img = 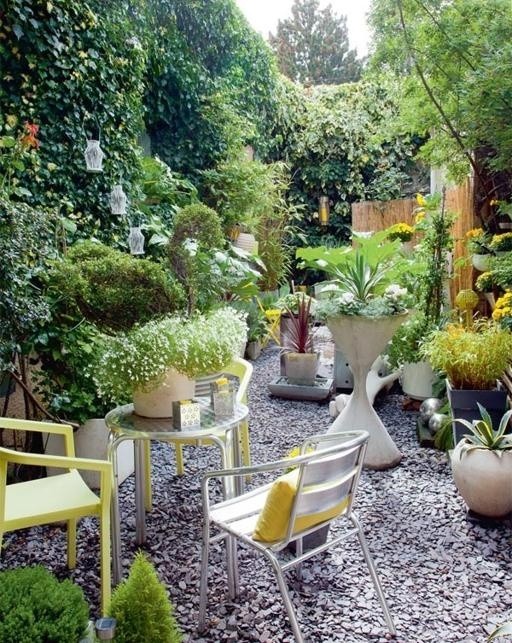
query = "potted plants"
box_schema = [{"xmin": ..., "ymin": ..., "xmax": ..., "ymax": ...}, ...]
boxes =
[
  {"xmin": 418, "ymin": 313, "xmax": 512, "ymax": 446},
  {"xmin": 381, "ymin": 319, "xmax": 440, "ymax": 401},
  {"xmin": 163, "ymin": 203, "xmax": 225, "ymax": 396},
  {"xmin": 93, "ymin": 307, "xmax": 231, "ymax": 418},
  {"xmin": 246, "ymin": 311, "xmax": 271, "ymax": 359},
  {"xmin": 467, "ymin": 234, "xmax": 492, "ymax": 271},
  {"xmin": 277, "ymin": 291, "xmax": 317, "ymax": 376},
  {"xmin": 41, "ymin": 325, "xmax": 134, "ymax": 489},
  {"xmin": 283, "ymin": 296, "xmax": 317, "ymax": 385},
  {"xmin": 452, "ymin": 401, "xmax": 512, "ymax": 518},
  {"xmin": 295, "ymin": 230, "xmax": 409, "ymax": 470},
  {"xmin": 232, "ymin": 209, "xmax": 262, "ymax": 251}
]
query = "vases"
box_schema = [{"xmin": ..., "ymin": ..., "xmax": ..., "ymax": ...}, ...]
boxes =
[{"xmin": 268, "ymin": 375, "xmax": 334, "ymax": 400}]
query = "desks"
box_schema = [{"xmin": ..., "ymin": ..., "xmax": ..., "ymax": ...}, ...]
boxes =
[{"xmin": 105, "ymin": 398, "xmax": 249, "ymax": 601}]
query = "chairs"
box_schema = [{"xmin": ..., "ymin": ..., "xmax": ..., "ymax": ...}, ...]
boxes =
[
  {"xmin": 198, "ymin": 431, "xmax": 396, "ymax": 643},
  {"xmin": 0, "ymin": 417, "xmax": 113, "ymax": 618},
  {"xmin": 144, "ymin": 357, "xmax": 254, "ymax": 511}
]
